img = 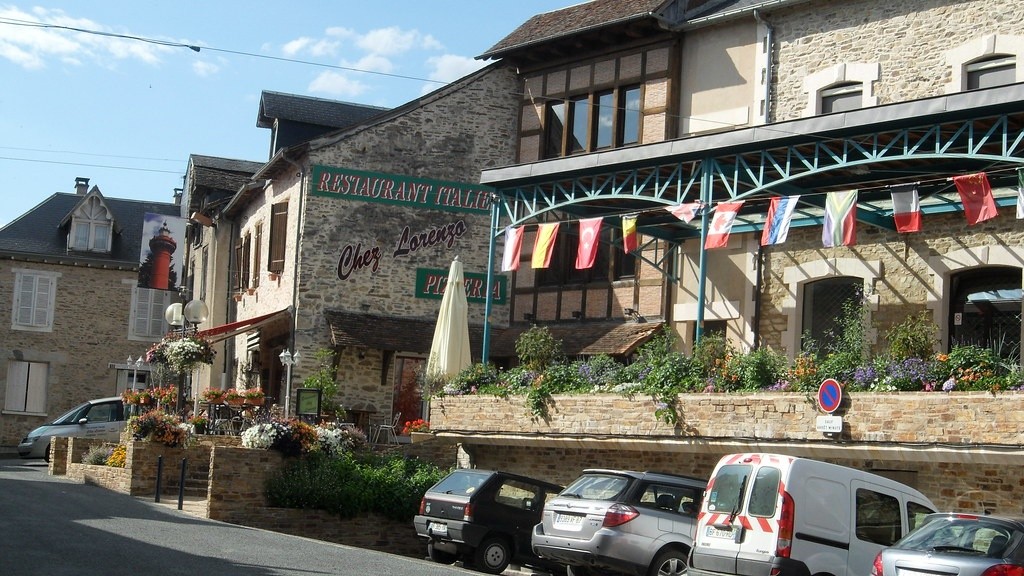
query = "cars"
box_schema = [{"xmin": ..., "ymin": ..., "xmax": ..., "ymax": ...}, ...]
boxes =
[
  {"xmin": 869, "ymin": 512, "xmax": 1024, "ymax": 576},
  {"xmin": 17, "ymin": 397, "xmax": 131, "ymax": 463},
  {"xmin": 414, "ymin": 469, "xmax": 566, "ymax": 575},
  {"xmin": 532, "ymin": 469, "xmax": 709, "ymax": 576}
]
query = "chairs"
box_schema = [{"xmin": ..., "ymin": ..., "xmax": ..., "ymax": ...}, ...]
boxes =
[
  {"xmin": 988, "ymin": 536, "xmax": 1008, "ymax": 554},
  {"xmin": 658, "ymin": 494, "xmax": 674, "ymax": 510},
  {"xmin": 372, "ymin": 412, "xmax": 402, "ymax": 445}
]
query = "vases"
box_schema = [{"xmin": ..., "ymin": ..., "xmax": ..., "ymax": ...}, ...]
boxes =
[
  {"xmin": 247, "ymin": 289, "xmax": 255, "ymax": 296},
  {"xmin": 228, "ymin": 399, "xmax": 244, "ymax": 405},
  {"xmin": 234, "ymin": 295, "xmax": 241, "ymax": 302},
  {"xmin": 195, "ymin": 424, "xmax": 204, "ymax": 434},
  {"xmin": 248, "ymin": 398, "xmax": 265, "ymax": 405},
  {"xmin": 268, "ymin": 274, "xmax": 277, "ymax": 280},
  {"xmin": 206, "ymin": 398, "xmax": 223, "ymax": 404},
  {"xmin": 140, "ymin": 397, "xmax": 149, "ymax": 404}
]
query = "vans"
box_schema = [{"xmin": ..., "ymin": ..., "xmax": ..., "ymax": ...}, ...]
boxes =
[{"xmin": 687, "ymin": 453, "xmax": 958, "ymax": 576}]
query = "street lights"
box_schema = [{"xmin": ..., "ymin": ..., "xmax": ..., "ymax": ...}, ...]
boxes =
[
  {"xmin": 127, "ymin": 355, "xmax": 144, "ymax": 393},
  {"xmin": 165, "ymin": 299, "xmax": 208, "ymax": 412},
  {"xmin": 279, "ymin": 348, "xmax": 302, "ymax": 418}
]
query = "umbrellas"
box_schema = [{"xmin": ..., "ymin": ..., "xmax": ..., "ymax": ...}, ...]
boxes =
[{"xmin": 425, "ymin": 255, "xmax": 471, "ymax": 377}]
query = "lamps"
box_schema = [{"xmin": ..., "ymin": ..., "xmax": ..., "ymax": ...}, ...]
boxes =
[
  {"xmin": 624, "ymin": 307, "xmax": 646, "ymax": 324},
  {"xmin": 191, "ymin": 212, "xmax": 215, "ymax": 228},
  {"xmin": 572, "ymin": 311, "xmax": 587, "ymax": 322},
  {"xmin": 524, "ymin": 313, "xmax": 536, "ymax": 324}
]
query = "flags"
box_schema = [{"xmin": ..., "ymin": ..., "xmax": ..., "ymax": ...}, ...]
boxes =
[
  {"xmin": 704, "ymin": 202, "xmax": 743, "ymax": 250},
  {"xmin": 953, "ymin": 172, "xmax": 998, "ymax": 226},
  {"xmin": 622, "ymin": 215, "xmax": 637, "ymax": 254},
  {"xmin": 575, "ymin": 217, "xmax": 602, "ymax": 269},
  {"xmin": 501, "ymin": 224, "xmax": 524, "ymax": 272},
  {"xmin": 890, "ymin": 184, "xmax": 922, "ymax": 234},
  {"xmin": 530, "ymin": 222, "xmax": 559, "ymax": 268},
  {"xmin": 1016, "ymin": 168, "xmax": 1024, "ymax": 219},
  {"xmin": 761, "ymin": 197, "xmax": 799, "ymax": 246},
  {"xmin": 663, "ymin": 203, "xmax": 705, "ymax": 224},
  {"xmin": 821, "ymin": 189, "xmax": 858, "ymax": 248}
]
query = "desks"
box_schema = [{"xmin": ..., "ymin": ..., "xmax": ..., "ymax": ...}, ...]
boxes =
[{"xmin": 346, "ymin": 409, "xmax": 376, "ymax": 443}]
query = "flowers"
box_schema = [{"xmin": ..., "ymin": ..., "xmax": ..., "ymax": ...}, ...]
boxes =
[
  {"xmin": 239, "ymin": 421, "xmax": 345, "ymax": 456},
  {"xmin": 121, "ymin": 384, "xmax": 208, "ymax": 447},
  {"xmin": 146, "ymin": 330, "xmax": 217, "ymax": 377},
  {"xmin": 81, "ymin": 443, "xmax": 127, "ymax": 468},
  {"xmin": 403, "ymin": 277, "xmax": 1024, "ymax": 437},
  {"xmin": 202, "ymin": 387, "xmax": 265, "ymax": 401}
]
